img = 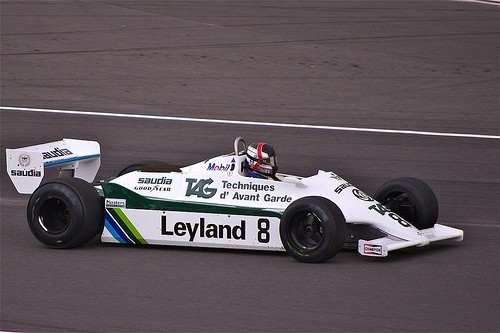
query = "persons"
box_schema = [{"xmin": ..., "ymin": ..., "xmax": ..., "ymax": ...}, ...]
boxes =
[{"xmin": 245, "ymin": 142, "xmax": 281, "ymax": 181}]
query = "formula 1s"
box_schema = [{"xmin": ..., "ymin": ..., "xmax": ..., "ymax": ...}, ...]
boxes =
[{"xmin": 5, "ymin": 136, "xmax": 465, "ymax": 265}]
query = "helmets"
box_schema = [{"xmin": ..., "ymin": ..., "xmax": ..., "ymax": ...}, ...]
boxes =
[{"xmin": 246, "ymin": 142, "xmax": 279, "ymax": 176}]
